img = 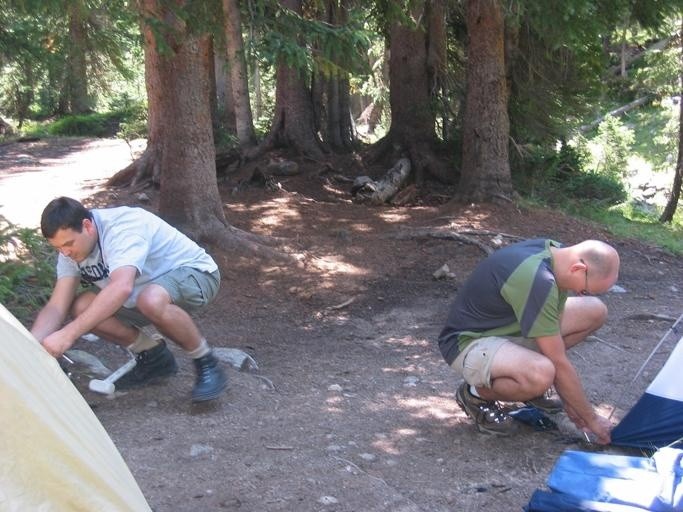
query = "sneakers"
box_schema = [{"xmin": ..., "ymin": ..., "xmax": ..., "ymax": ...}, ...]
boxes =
[
  {"xmin": 113, "ymin": 340, "xmax": 178, "ymax": 392},
  {"xmin": 455, "ymin": 381, "xmax": 520, "ymax": 436},
  {"xmin": 524, "ymin": 392, "xmax": 564, "ymax": 414},
  {"xmin": 190, "ymin": 350, "xmax": 228, "ymax": 401}
]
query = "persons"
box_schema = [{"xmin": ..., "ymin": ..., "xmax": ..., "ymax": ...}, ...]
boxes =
[
  {"xmin": 28, "ymin": 197, "xmax": 232, "ymax": 403},
  {"xmin": 438, "ymin": 239, "xmax": 620, "ymax": 446}
]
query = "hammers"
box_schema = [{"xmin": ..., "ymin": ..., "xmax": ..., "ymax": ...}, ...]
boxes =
[{"xmin": 80, "ymin": 359, "xmax": 138, "ymax": 395}]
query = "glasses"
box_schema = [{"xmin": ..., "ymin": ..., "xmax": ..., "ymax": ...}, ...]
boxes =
[{"xmin": 580, "ymin": 259, "xmax": 589, "ymax": 296}]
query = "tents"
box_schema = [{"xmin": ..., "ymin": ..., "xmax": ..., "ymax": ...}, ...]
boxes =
[
  {"xmin": 0, "ymin": 294, "xmax": 153, "ymax": 512},
  {"xmin": 597, "ymin": 336, "xmax": 683, "ymax": 449}
]
